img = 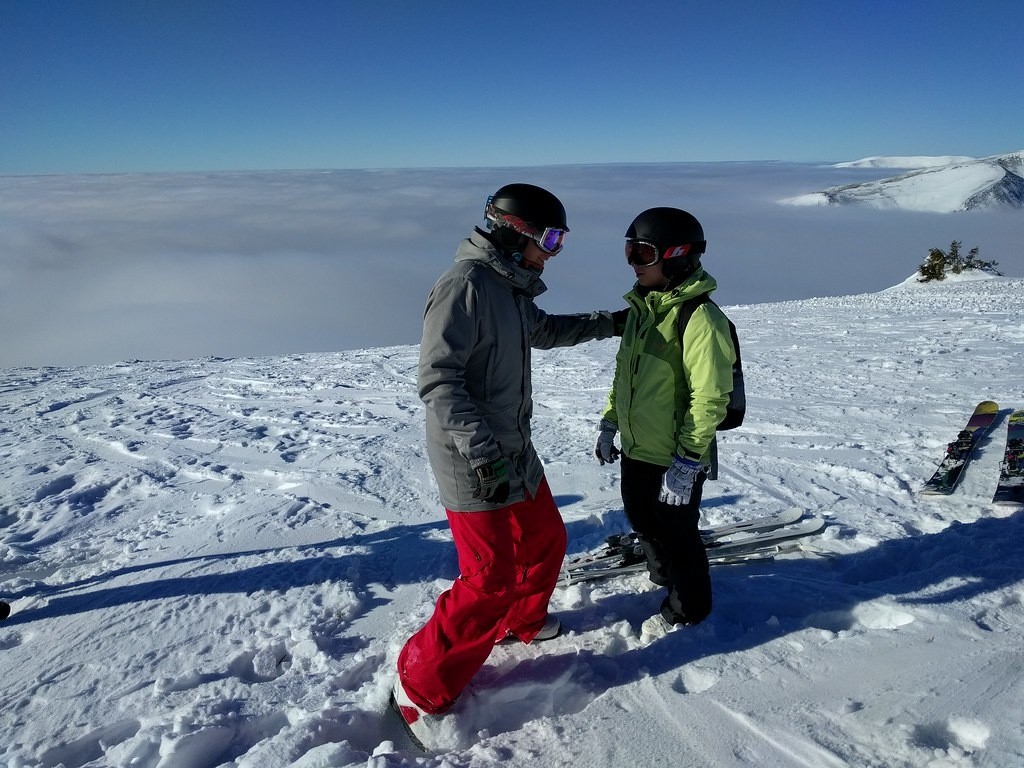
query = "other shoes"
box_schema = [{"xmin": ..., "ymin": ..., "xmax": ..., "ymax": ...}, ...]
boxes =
[
  {"xmin": 635, "ymin": 579, "xmax": 663, "ymax": 594},
  {"xmin": 390, "ymin": 673, "xmax": 444, "ymax": 752},
  {"xmin": 637, "ymin": 610, "xmax": 674, "ymax": 643},
  {"xmin": 495, "ymin": 614, "xmax": 561, "ymax": 645}
]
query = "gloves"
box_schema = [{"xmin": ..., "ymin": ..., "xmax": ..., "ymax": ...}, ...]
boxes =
[
  {"xmin": 473, "ymin": 457, "xmax": 511, "ymax": 505},
  {"xmin": 611, "ymin": 307, "xmax": 632, "ymax": 337},
  {"xmin": 594, "ymin": 419, "xmax": 620, "ymax": 466},
  {"xmin": 658, "ymin": 452, "xmax": 702, "ymax": 506}
]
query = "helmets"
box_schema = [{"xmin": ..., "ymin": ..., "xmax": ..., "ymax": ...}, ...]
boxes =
[
  {"xmin": 487, "ymin": 183, "xmax": 570, "ymax": 252},
  {"xmin": 624, "ymin": 207, "xmax": 704, "ymax": 281}
]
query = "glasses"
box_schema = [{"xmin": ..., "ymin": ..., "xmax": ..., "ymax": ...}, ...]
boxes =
[
  {"xmin": 536, "ymin": 226, "xmax": 563, "ymax": 255},
  {"xmin": 625, "ymin": 239, "xmax": 661, "ymax": 267}
]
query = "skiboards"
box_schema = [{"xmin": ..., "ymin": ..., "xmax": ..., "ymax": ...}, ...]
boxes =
[
  {"xmin": 557, "ymin": 507, "xmax": 826, "ymax": 585},
  {"xmin": 916, "ymin": 400, "xmax": 1024, "ymax": 505}
]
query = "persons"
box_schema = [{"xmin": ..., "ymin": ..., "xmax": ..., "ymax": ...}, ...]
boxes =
[
  {"xmin": 392, "ymin": 184, "xmax": 632, "ymax": 753},
  {"xmin": 595, "ymin": 207, "xmax": 736, "ymax": 638}
]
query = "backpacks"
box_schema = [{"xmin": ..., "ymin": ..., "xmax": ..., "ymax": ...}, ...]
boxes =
[{"xmin": 679, "ymin": 295, "xmax": 746, "ymax": 431}]
